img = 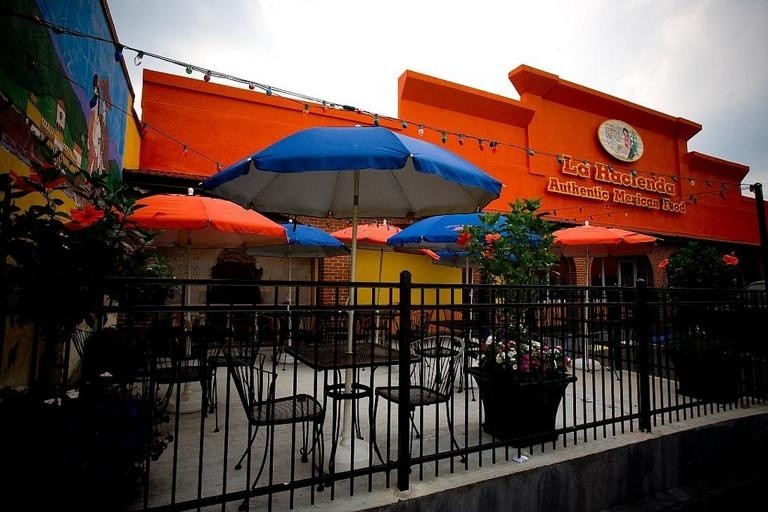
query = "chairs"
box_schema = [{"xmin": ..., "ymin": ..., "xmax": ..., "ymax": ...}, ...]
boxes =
[
  {"xmin": 67, "ymin": 319, "xmax": 265, "ymax": 415},
  {"xmin": 220, "ymin": 308, "xmax": 491, "ymax": 490}
]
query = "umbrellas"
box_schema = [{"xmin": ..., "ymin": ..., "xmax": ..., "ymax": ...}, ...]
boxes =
[
  {"xmin": 63, "ymin": 188, "xmax": 289, "ymax": 317},
  {"xmin": 386, "ymin": 210, "xmax": 544, "ymax": 283},
  {"xmin": 193, "ymin": 124, "xmax": 501, "ymax": 352},
  {"xmin": 537, "ymin": 225, "xmax": 662, "ymax": 357},
  {"xmin": 659, "ymin": 252, "xmax": 741, "ymax": 269},
  {"xmin": 431, "ymin": 249, "xmax": 525, "ymax": 284},
  {"xmin": 328, "ymin": 222, "xmax": 440, "ymax": 309},
  {"xmin": 223, "ymin": 220, "xmax": 350, "ymax": 305}
]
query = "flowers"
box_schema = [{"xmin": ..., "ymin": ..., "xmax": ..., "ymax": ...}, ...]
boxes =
[
  {"xmin": 451, "ymin": 197, "xmax": 570, "ymax": 381},
  {"xmin": 0, "ymin": 151, "xmax": 156, "ymax": 281},
  {"xmin": 50, "ymin": 370, "xmax": 176, "ymax": 470},
  {"xmin": 657, "ymin": 245, "xmax": 749, "ymax": 348}
]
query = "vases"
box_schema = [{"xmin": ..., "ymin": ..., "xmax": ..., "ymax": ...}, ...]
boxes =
[
  {"xmin": 665, "ymin": 339, "xmax": 750, "ymax": 405},
  {"xmin": 465, "ymin": 361, "xmax": 580, "ymax": 448}
]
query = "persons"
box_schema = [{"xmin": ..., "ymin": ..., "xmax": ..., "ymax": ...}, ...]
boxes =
[{"xmin": 622, "ymin": 128, "xmax": 634, "ymax": 153}]
